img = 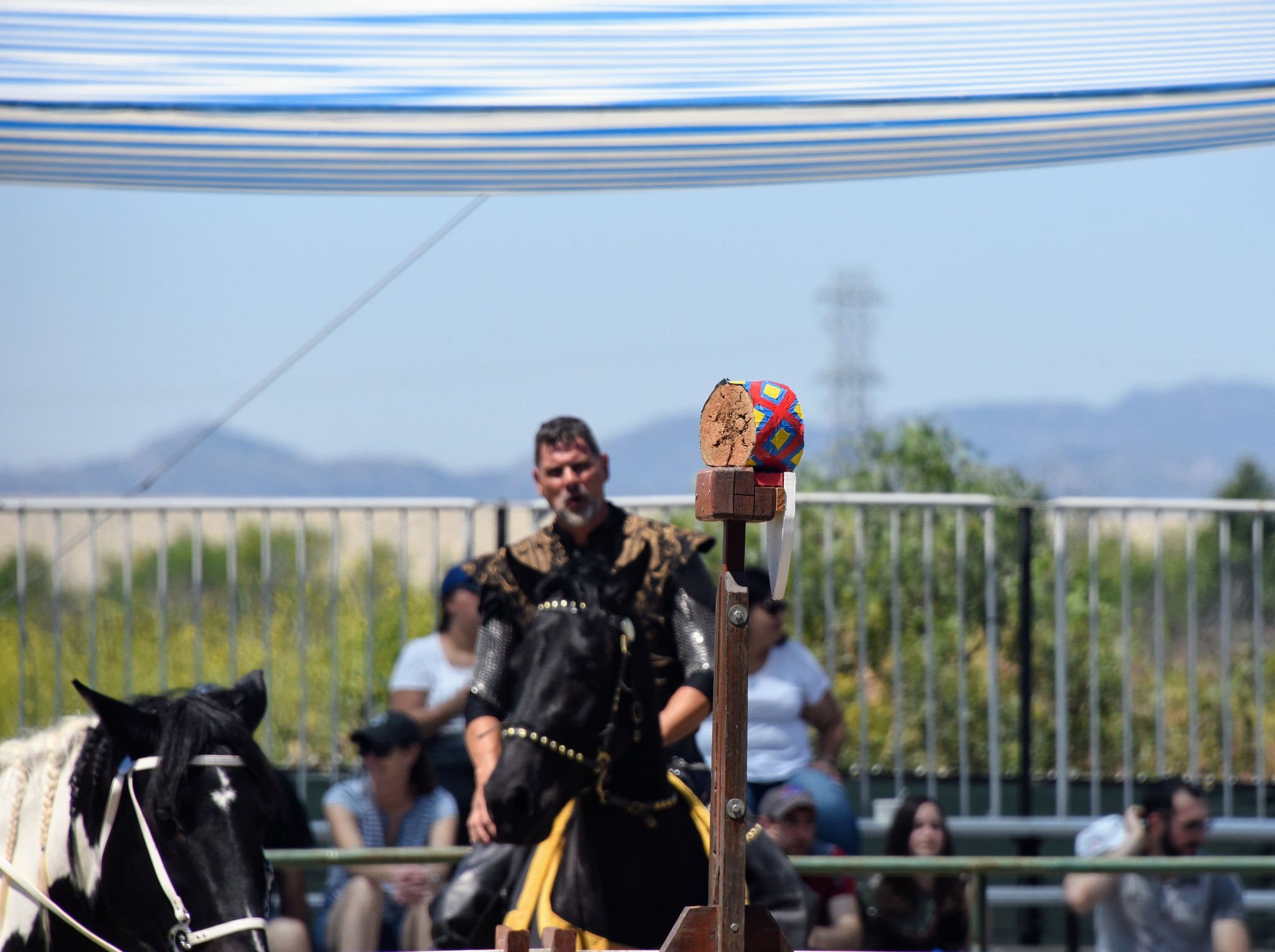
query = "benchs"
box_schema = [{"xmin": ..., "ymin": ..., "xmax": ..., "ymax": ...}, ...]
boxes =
[{"xmin": 306, "ymin": 815, "xmax": 1274, "ymax": 915}]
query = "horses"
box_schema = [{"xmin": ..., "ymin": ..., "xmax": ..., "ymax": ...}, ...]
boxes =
[
  {"xmin": 480, "ymin": 547, "xmax": 795, "ymax": 952},
  {"xmin": 0, "ymin": 668, "xmax": 275, "ymax": 952}
]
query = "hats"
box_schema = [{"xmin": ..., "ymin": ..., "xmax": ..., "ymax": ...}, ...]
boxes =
[
  {"xmin": 757, "ymin": 785, "xmax": 821, "ymax": 820},
  {"xmin": 441, "ymin": 561, "xmax": 478, "ymax": 596},
  {"xmin": 744, "ymin": 566, "xmax": 785, "ymax": 614},
  {"xmin": 348, "ymin": 710, "xmax": 423, "ymax": 749}
]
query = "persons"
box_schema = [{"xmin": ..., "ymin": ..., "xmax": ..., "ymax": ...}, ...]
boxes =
[
  {"xmin": 428, "ymin": 416, "xmax": 809, "ymax": 952},
  {"xmin": 690, "ymin": 563, "xmax": 861, "ymax": 861},
  {"xmin": 862, "ymin": 794, "xmax": 971, "ymax": 952},
  {"xmin": 1062, "ymin": 774, "xmax": 1255, "ymax": 952},
  {"xmin": 753, "ymin": 784, "xmax": 862, "ymax": 952},
  {"xmin": 261, "ymin": 554, "xmax": 488, "ymax": 952}
]
